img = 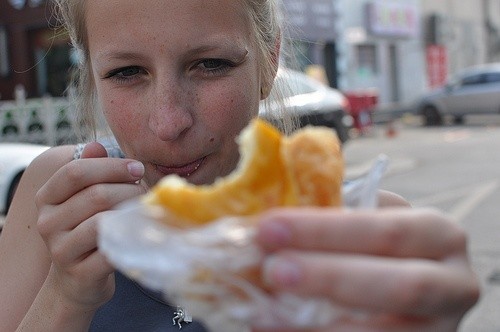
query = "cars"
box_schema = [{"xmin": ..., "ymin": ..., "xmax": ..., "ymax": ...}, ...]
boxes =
[
  {"xmin": 0, "ymin": 142, "xmax": 55, "ymax": 217},
  {"xmin": 415, "ymin": 62, "xmax": 500, "ymax": 127},
  {"xmin": 259, "ymin": 66, "xmax": 348, "ymax": 143}
]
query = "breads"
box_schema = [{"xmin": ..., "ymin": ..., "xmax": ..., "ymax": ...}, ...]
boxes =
[{"xmin": 146, "ymin": 118, "xmax": 343, "ymax": 289}]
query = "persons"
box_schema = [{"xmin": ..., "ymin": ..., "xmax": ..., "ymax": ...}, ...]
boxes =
[{"xmin": 0, "ymin": 0, "xmax": 481, "ymax": 332}]
